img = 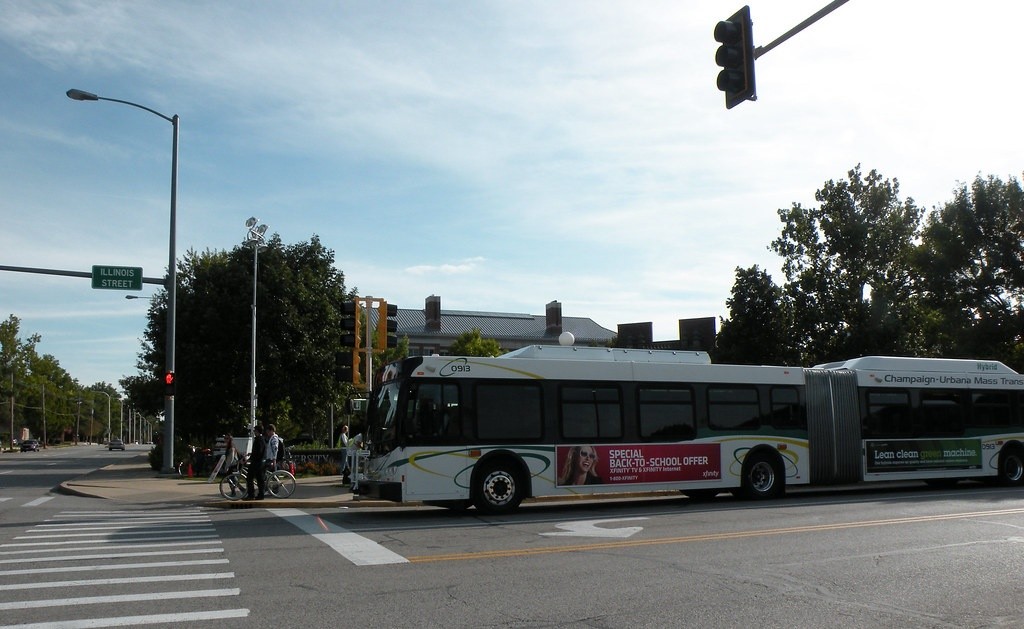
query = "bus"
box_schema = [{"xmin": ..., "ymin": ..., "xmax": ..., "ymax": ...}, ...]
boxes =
[{"xmin": 341, "ymin": 344, "xmax": 1024, "ymax": 506}]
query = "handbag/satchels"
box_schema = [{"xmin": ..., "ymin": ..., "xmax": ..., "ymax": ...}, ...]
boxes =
[{"xmin": 239, "ymin": 454, "xmax": 247, "ymax": 465}]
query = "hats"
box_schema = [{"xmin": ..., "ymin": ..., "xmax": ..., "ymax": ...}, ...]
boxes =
[
  {"xmin": 354, "ymin": 433, "xmax": 363, "ymax": 443},
  {"xmin": 254, "ymin": 425, "xmax": 264, "ymax": 433}
]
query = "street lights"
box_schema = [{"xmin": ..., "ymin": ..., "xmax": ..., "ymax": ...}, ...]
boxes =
[
  {"xmin": 245, "ymin": 217, "xmax": 270, "ymax": 437},
  {"xmin": 66, "ymin": 88, "xmax": 175, "ymax": 476},
  {"xmin": 90, "ymin": 390, "xmax": 110, "ymax": 442}
]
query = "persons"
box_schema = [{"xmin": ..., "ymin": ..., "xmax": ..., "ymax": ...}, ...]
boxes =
[
  {"xmin": 343, "ymin": 433, "xmax": 362, "ymax": 484},
  {"xmin": 241, "ymin": 425, "xmax": 266, "ymax": 501},
  {"xmin": 557, "ymin": 446, "xmax": 604, "ymax": 486},
  {"xmin": 264, "ymin": 424, "xmax": 279, "ymax": 496},
  {"xmin": 224, "ymin": 432, "xmax": 246, "ymax": 497},
  {"xmin": 338, "ymin": 425, "xmax": 349, "ymax": 475}
]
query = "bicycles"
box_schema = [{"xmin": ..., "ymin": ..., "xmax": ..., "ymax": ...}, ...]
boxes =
[
  {"xmin": 218, "ymin": 453, "xmax": 297, "ymax": 502},
  {"xmin": 178, "ymin": 444, "xmax": 230, "ymax": 478}
]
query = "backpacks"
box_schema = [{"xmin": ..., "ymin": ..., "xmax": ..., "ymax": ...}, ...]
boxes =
[{"xmin": 278, "ymin": 439, "xmax": 284, "ymax": 460}]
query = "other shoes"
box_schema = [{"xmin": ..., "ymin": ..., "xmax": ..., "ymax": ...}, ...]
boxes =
[
  {"xmin": 229, "ymin": 492, "xmax": 236, "ymax": 496},
  {"xmin": 241, "ymin": 489, "xmax": 246, "ymax": 496}
]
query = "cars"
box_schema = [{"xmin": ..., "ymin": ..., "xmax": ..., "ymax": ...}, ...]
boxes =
[
  {"xmin": 108, "ymin": 439, "xmax": 127, "ymax": 451},
  {"xmin": 19, "ymin": 439, "xmax": 41, "ymax": 453}
]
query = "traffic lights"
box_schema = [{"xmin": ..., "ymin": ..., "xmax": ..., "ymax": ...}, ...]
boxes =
[
  {"xmin": 340, "ymin": 297, "xmax": 360, "ymax": 348},
  {"xmin": 164, "ymin": 371, "xmax": 177, "ymax": 397},
  {"xmin": 377, "ymin": 300, "xmax": 399, "ymax": 352},
  {"xmin": 713, "ymin": 5, "xmax": 757, "ymax": 109},
  {"xmin": 335, "ymin": 349, "xmax": 360, "ymax": 386}
]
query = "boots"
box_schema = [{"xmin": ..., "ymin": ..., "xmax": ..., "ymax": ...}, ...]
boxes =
[
  {"xmin": 255, "ymin": 481, "xmax": 266, "ymax": 500},
  {"xmin": 243, "ymin": 481, "xmax": 255, "ymax": 501}
]
